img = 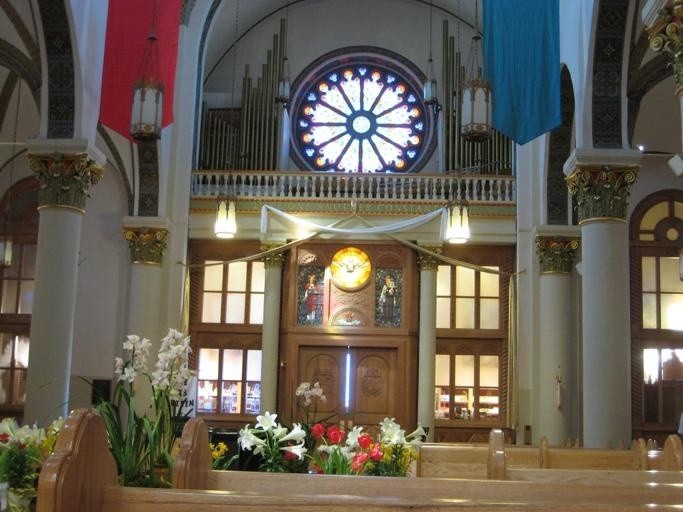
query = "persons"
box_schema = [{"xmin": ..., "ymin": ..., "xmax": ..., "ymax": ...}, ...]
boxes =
[
  {"xmin": 379, "ymin": 274, "xmax": 397, "ymax": 326},
  {"xmin": 302, "ymin": 274, "xmax": 320, "ymax": 321}
]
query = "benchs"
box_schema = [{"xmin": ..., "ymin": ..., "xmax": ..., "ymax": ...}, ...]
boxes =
[{"xmin": 38, "ymin": 408, "xmax": 682, "ymax": 512}]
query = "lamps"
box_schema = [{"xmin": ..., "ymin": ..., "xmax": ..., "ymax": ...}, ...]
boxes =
[
  {"xmin": 441, "ymin": 1, "xmax": 471, "ymax": 245},
  {"xmin": 214, "ymin": 2, "xmax": 240, "ymax": 242},
  {"xmin": 274, "ymin": 0, "xmax": 292, "ymax": 108},
  {"xmin": 459, "ymin": 0, "xmax": 493, "ymax": 144},
  {"xmin": 127, "ymin": 0, "xmax": 164, "ymax": 143},
  {"xmin": 422, "ymin": 0, "xmax": 438, "ymax": 105}
]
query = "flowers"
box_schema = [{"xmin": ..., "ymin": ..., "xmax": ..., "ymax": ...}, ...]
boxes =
[{"xmin": 0, "ymin": 413, "xmax": 47, "ymax": 488}]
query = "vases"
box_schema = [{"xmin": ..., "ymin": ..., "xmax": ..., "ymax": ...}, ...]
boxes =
[{"xmin": 4, "ymin": 484, "xmax": 34, "ymax": 510}]
